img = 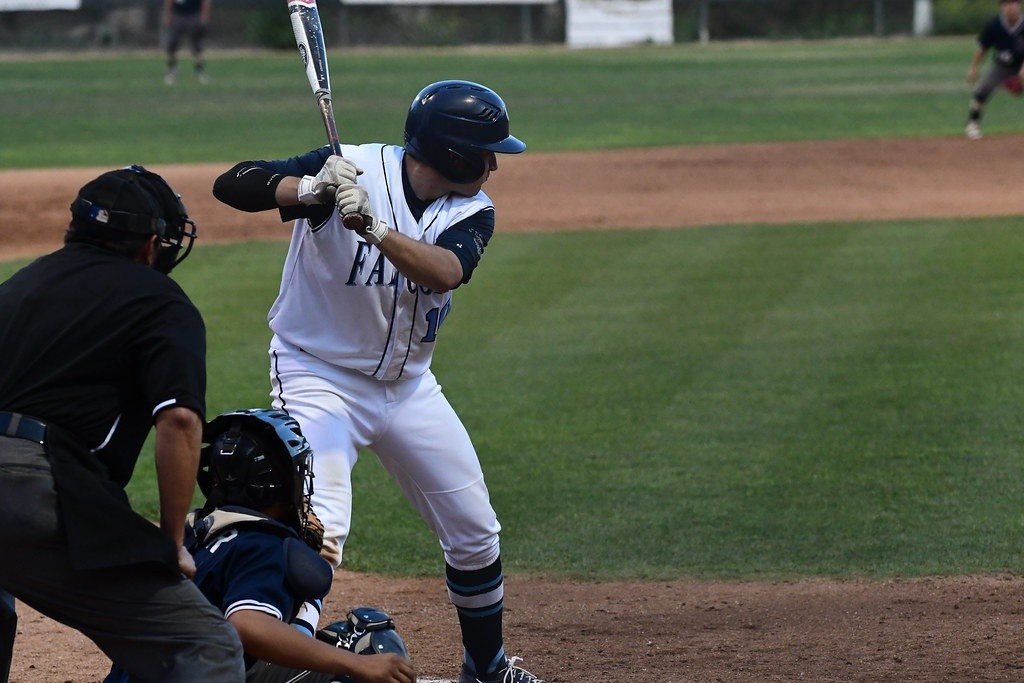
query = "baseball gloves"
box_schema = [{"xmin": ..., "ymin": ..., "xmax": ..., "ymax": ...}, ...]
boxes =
[
  {"xmin": 297, "ymin": 492, "xmax": 325, "ymax": 555},
  {"xmin": 1003, "ymin": 74, "xmax": 1023, "ymax": 95}
]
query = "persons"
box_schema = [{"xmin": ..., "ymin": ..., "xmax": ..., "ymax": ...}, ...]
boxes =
[
  {"xmin": 0, "ymin": 163, "xmax": 250, "ymax": 683},
  {"xmin": 160, "ymin": 0, "xmax": 214, "ymax": 86},
  {"xmin": 962, "ymin": 0, "xmax": 1024, "ymax": 142},
  {"xmin": 101, "ymin": 406, "xmax": 419, "ymax": 682},
  {"xmin": 213, "ymin": 79, "xmax": 549, "ymax": 683}
]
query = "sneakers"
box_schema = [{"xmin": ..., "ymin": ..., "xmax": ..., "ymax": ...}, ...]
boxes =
[{"xmin": 459, "ymin": 656, "xmax": 547, "ymax": 683}]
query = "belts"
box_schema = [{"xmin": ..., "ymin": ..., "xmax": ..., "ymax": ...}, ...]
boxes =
[{"xmin": 0, "ymin": 411, "xmax": 45, "ymax": 445}]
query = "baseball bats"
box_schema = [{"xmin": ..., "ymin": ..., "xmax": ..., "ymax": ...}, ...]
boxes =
[{"xmin": 285, "ymin": 0, "xmax": 363, "ymax": 230}]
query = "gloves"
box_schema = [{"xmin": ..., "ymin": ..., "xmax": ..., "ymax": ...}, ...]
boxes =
[
  {"xmin": 298, "ymin": 154, "xmax": 363, "ymax": 208},
  {"xmin": 335, "ymin": 184, "xmax": 388, "ymax": 247}
]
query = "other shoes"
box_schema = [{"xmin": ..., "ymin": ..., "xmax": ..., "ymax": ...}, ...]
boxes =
[{"xmin": 965, "ymin": 122, "xmax": 982, "ymax": 140}]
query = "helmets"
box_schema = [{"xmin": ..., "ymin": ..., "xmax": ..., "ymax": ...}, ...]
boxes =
[
  {"xmin": 199, "ymin": 408, "xmax": 315, "ymax": 514},
  {"xmin": 405, "ymin": 80, "xmax": 527, "ymax": 185},
  {"xmin": 68, "ymin": 163, "xmax": 197, "ymax": 273}
]
